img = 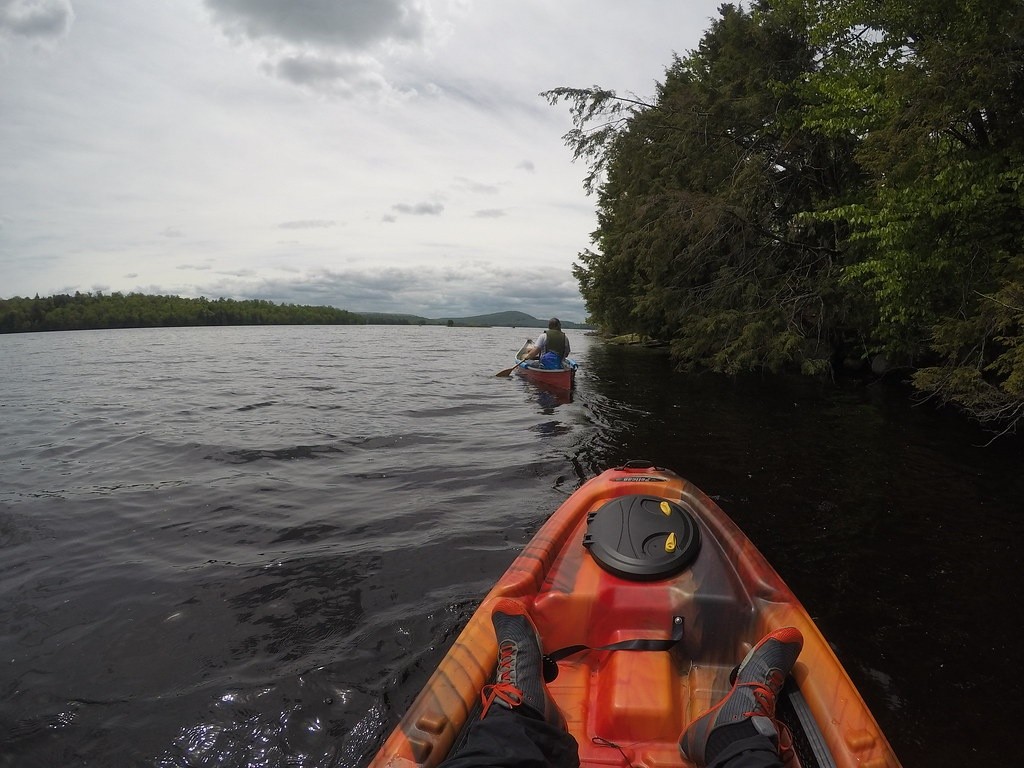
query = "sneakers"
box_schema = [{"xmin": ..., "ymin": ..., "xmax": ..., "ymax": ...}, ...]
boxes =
[
  {"xmin": 479, "ymin": 599, "xmax": 568, "ymax": 734},
  {"xmin": 678, "ymin": 626, "xmax": 804, "ymax": 767}
]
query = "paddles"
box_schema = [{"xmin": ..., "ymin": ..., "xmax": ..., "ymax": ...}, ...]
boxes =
[{"xmin": 495, "ymin": 359, "xmax": 527, "ymax": 378}]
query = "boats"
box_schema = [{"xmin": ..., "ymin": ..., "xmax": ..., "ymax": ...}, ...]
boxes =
[{"xmin": 516, "ymin": 337, "xmax": 579, "ymax": 394}]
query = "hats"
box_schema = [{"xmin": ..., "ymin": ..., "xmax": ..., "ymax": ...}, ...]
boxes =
[{"xmin": 549, "ymin": 317, "xmax": 561, "ymax": 329}]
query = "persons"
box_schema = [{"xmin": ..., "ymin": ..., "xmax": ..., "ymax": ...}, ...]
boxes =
[{"xmin": 528, "ymin": 318, "xmax": 571, "ymax": 364}]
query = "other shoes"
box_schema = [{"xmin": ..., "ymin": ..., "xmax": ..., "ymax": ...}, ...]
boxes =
[{"xmin": 528, "ymin": 349, "xmax": 539, "ymax": 355}]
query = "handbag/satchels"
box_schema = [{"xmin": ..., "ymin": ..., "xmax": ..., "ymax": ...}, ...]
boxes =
[{"xmin": 540, "ymin": 351, "xmax": 564, "ymax": 370}]
